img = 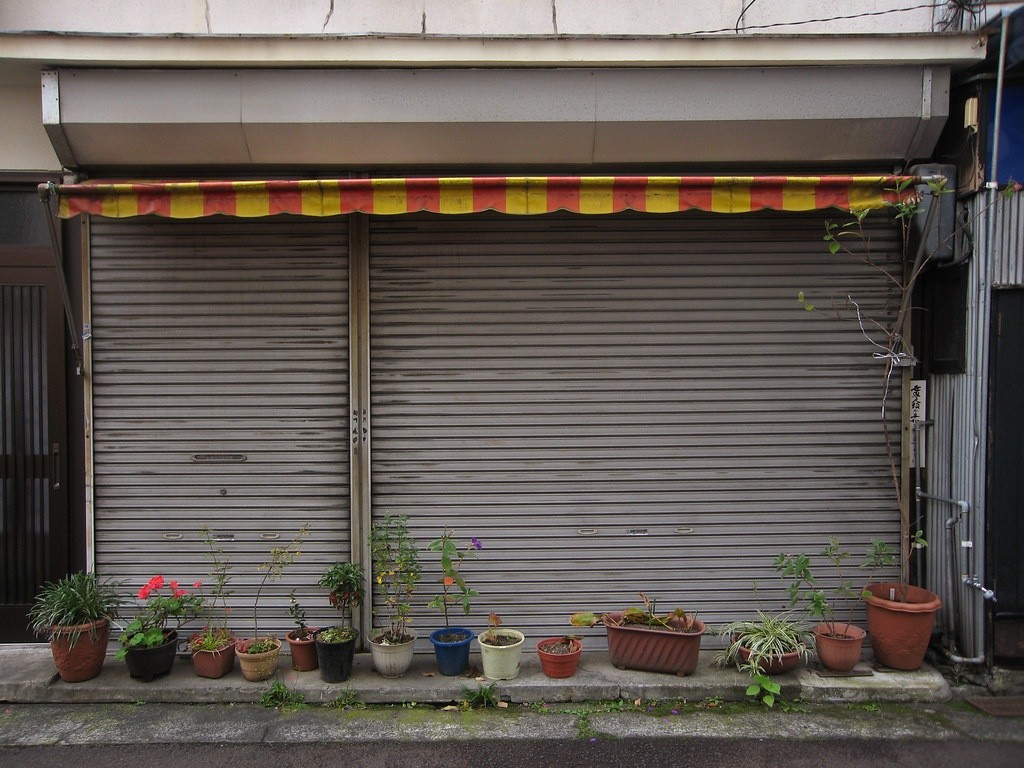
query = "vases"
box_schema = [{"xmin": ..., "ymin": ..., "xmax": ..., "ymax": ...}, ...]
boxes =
[
  {"xmin": 430, "ymin": 627, "xmax": 474, "ymax": 678},
  {"xmin": 121, "ymin": 629, "xmax": 179, "ymax": 683},
  {"xmin": 234, "ymin": 637, "xmax": 283, "ymax": 683},
  {"xmin": 190, "ymin": 639, "xmax": 239, "ymax": 678}
]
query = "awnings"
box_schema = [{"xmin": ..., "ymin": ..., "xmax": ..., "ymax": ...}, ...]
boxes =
[{"xmin": 56, "ymin": 172, "xmax": 920, "ymax": 219}]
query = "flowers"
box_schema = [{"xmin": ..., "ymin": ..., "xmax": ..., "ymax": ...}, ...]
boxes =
[
  {"xmin": 114, "ymin": 574, "xmax": 205, "ymax": 661},
  {"xmin": 237, "ymin": 520, "xmax": 314, "ymax": 654},
  {"xmin": 187, "ymin": 523, "xmax": 236, "ymax": 658},
  {"xmin": 424, "ymin": 524, "xmax": 483, "ymax": 635}
]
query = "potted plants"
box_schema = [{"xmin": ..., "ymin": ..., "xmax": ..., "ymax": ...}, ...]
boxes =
[
  {"xmin": 286, "ymin": 586, "xmax": 322, "ymax": 670},
  {"xmin": 863, "ymin": 528, "xmax": 943, "ymax": 672},
  {"xmin": 536, "ymin": 633, "xmax": 586, "ymax": 679},
  {"xmin": 771, "ymin": 534, "xmax": 898, "ymax": 675},
  {"xmin": 476, "ymin": 608, "xmax": 525, "ymax": 681},
  {"xmin": 313, "ymin": 559, "xmax": 369, "ymax": 684},
  {"xmin": 568, "ymin": 592, "xmax": 706, "ymax": 677},
  {"xmin": 24, "ymin": 563, "xmax": 140, "ymax": 683},
  {"xmin": 705, "ymin": 577, "xmax": 817, "ymax": 677},
  {"xmin": 366, "ymin": 508, "xmax": 423, "ymax": 680}
]
